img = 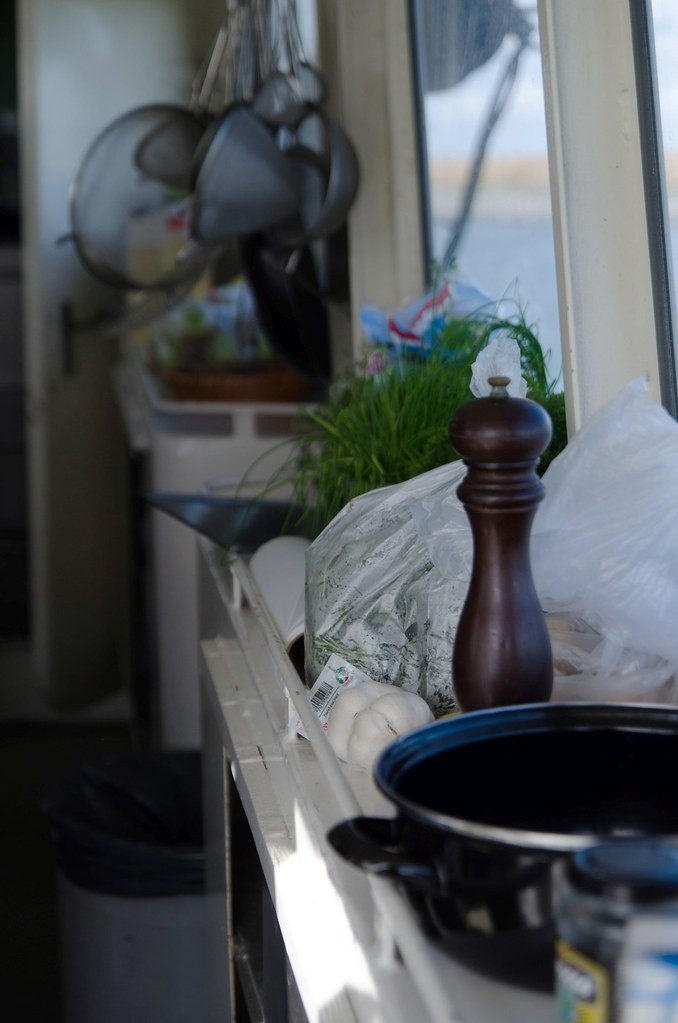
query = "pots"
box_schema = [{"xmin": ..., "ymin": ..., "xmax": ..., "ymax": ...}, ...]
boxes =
[{"xmin": 326, "ymin": 700, "xmax": 678, "ymax": 993}]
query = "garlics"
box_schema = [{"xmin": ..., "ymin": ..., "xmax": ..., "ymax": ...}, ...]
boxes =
[{"xmin": 327, "ymin": 680, "xmax": 435, "ymax": 773}]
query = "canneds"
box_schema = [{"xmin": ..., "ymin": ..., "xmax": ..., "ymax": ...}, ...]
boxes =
[{"xmin": 551, "ymin": 839, "xmax": 678, "ymax": 1023}]
